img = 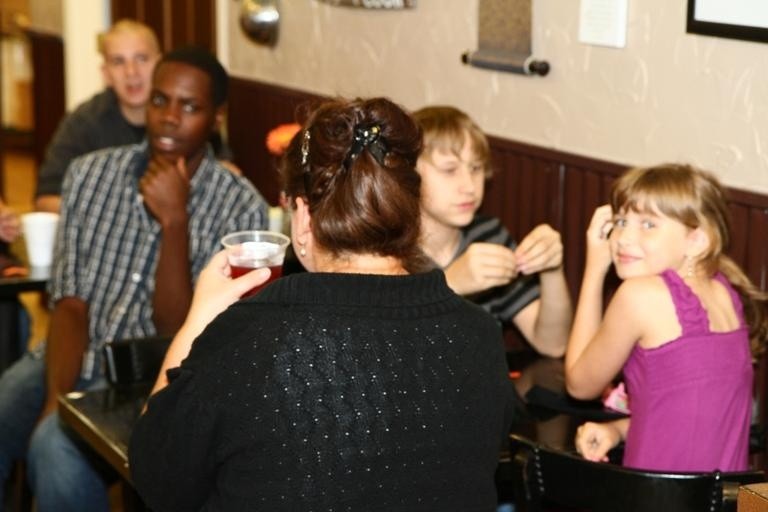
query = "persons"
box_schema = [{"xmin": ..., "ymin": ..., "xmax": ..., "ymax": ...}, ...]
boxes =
[
  {"xmin": 31, "ymin": 17, "xmax": 164, "ymax": 212},
  {"xmin": 129, "ymin": 96, "xmax": 519, "ymax": 512},
  {"xmin": 1, "ymin": 45, "xmax": 271, "ymax": 511},
  {"xmin": 562, "ymin": 160, "xmax": 767, "ymax": 476},
  {"xmin": 408, "ymin": 105, "xmax": 574, "ymax": 358},
  {"xmin": 2, "ymin": 203, "xmax": 31, "ymax": 368}
]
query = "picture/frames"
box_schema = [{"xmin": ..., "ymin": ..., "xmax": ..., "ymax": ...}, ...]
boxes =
[{"xmin": 686, "ymin": 0, "xmax": 768, "ymax": 45}]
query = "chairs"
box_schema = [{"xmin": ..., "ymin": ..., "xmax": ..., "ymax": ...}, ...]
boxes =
[{"xmin": 506, "ymin": 433, "xmax": 723, "ymax": 511}]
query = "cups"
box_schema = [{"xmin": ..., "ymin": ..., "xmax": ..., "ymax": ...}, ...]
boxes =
[
  {"xmin": 19, "ymin": 209, "xmax": 61, "ymax": 268},
  {"xmin": 219, "ymin": 232, "xmax": 291, "ymax": 297}
]
